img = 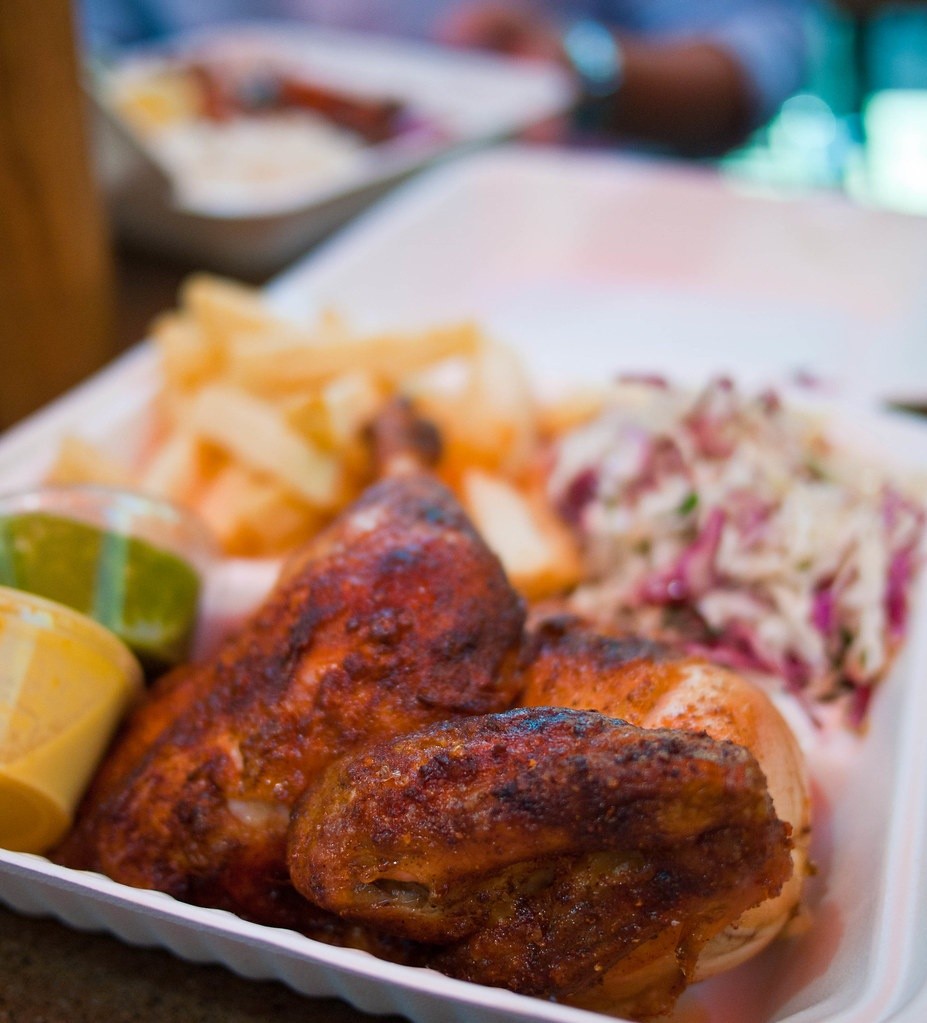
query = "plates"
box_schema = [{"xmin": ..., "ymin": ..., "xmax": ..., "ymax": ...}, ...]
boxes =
[
  {"xmin": 81, "ymin": 21, "xmax": 570, "ymax": 278},
  {"xmin": 1, "ymin": 148, "xmax": 922, "ymax": 1021}
]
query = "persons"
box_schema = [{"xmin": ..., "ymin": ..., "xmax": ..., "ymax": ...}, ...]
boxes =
[{"xmin": 74, "ymin": 0, "xmax": 841, "ymax": 164}]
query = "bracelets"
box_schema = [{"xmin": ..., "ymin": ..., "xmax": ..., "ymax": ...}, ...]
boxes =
[{"xmin": 561, "ymin": 26, "xmax": 619, "ymax": 124}]
